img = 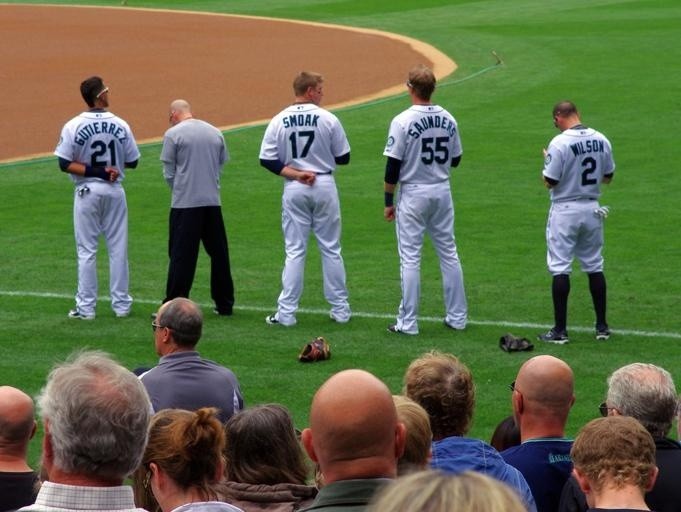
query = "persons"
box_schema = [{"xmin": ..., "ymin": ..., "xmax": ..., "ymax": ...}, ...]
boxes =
[
  {"xmin": 257, "ymin": 70, "xmax": 353, "ymax": 325},
  {"xmin": 541, "ymin": 100, "xmax": 618, "ymax": 343},
  {"xmin": 380, "ymin": 66, "xmax": 468, "ymax": 335},
  {"xmin": 54, "ymin": 75, "xmax": 140, "ymax": 319},
  {"xmin": 159, "ymin": 98, "xmax": 236, "ymax": 316},
  {"xmin": 137, "ymin": 297, "xmax": 244, "ymax": 421}
]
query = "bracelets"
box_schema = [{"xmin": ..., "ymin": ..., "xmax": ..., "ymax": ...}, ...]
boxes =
[{"xmin": 384, "ymin": 191, "xmax": 394, "ymax": 208}]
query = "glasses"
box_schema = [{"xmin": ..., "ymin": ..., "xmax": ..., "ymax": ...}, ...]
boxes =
[
  {"xmin": 551, "ymin": 114, "xmax": 566, "ymax": 128},
  {"xmin": 148, "ymin": 320, "xmax": 167, "ymax": 332},
  {"xmin": 166, "ymin": 112, "xmax": 175, "ymax": 124},
  {"xmin": 402, "ymin": 80, "xmax": 414, "ymax": 95},
  {"xmin": 508, "ymin": 381, "xmax": 519, "ymax": 399},
  {"xmin": 95, "ymin": 81, "xmax": 112, "ymax": 101},
  {"xmin": 140, "ymin": 464, "xmax": 156, "ymax": 500},
  {"xmin": 596, "ymin": 402, "xmax": 624, "ymax": 417}
]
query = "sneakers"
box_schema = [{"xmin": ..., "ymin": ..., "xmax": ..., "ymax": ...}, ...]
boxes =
[
  {"xmin": 149, "ymin": 309, "xmax": 160, "ymax": 320},
  {"xmin": 263, "ymin": 312, "xmax": 297, "ymax": 327},
  {"xmin": 115, "ymin": 308, "xmax": 132, "ymax": 320},
  {"xmin": 63, "ymin": 305, "xmax": 98, "ymax": 323},
  {"xmin": 384, "ymin": 319, "xmax": 421, "ymax": 338},
  {"xmin": 327, "ymin": 307, "xmax": 353, "ymax": 324},
  {"xmin": 440, "ymin": 315, "xmax": 467, "ymax": 332},
  {"xmin": 535, "ymin": 326, "xmax": 570, "ymax": 348},
  {"xmin": 211, "ymin": 306, "xmax": 233, "ymax": 318},
  {"xmin": 593, "ymin": 321, "xmax": 611, "ymax": 343}
]
